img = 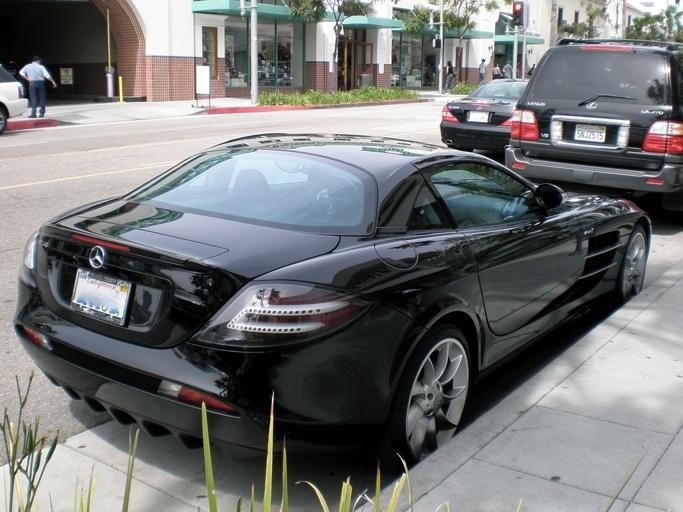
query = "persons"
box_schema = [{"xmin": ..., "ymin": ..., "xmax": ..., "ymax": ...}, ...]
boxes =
[
  {"xmin": 529, "ymin": 63, "xmax": 537, "ymax": 77},
  {"xmin": 492, "ymin": 62, "xmax": 503, "ymax": 79},
  {"xmin": 503, "ymin": 60, "xmax": 513, "ymax": 79},
  {"xmin": 478, "ymin": 58, "xmax": 487, "ymax": 82},
  {"xmin": 517, "ymin": 61, "xmax": 520, "ymax": 78},
  {"xmin": 18, "ymin": 54, "xmax": 57, "ymax": 118},
  {"xmin": 444, "ymin": 61, "xmax": 456, "ymax": 90}
]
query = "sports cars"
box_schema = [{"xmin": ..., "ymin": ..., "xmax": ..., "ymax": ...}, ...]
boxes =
[{"xmin": 11, "ymin": 128, "xmax": 654, "ymax": 480}]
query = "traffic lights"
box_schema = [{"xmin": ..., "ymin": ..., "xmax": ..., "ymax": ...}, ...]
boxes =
[{"xmin": 511, "ymin": 0, "xmax": 524, "ymax": 80}]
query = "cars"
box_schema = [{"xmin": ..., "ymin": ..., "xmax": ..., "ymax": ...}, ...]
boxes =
[
  {"xmin": 502, "ymin": 35, "xmax": 683, "ymax": 215},
  {"xmin": 0, "ymin": 63, "xmax": 30, "ymax": 134},
  {"xmin": 438, "ymin": 75, "xmax": 530, "ymax": 154}
]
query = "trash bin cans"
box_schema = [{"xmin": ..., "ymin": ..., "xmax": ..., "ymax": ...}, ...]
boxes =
[{"xmin": 359, "ymin": 74, "xmax": 373, "ymax": 88}]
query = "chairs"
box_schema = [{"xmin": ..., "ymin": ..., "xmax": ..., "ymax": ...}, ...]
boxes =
[
  {"xmin": 230, "ymin": 170, "xmax": 275, "ymax": 207},
  {"xmin": 404, "ymin": 75, "xmax": 422, "ymax": 91}
]
